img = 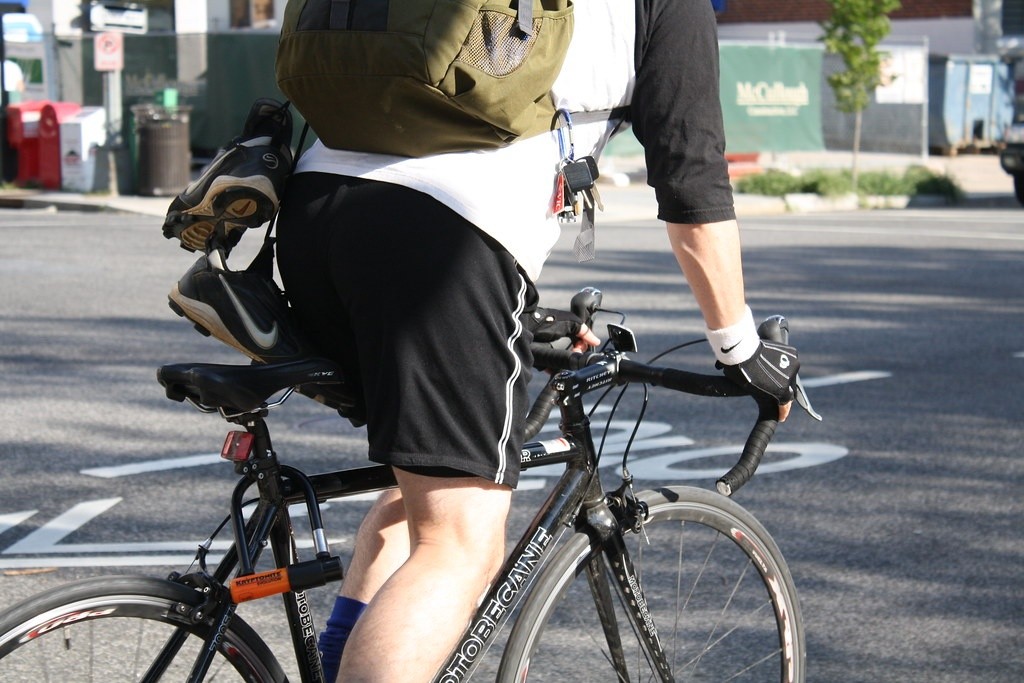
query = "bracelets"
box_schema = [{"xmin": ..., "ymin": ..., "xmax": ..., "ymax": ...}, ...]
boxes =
[{"xmin": 704, "ymin": 304, "xmax": 759, "ymax": 365}]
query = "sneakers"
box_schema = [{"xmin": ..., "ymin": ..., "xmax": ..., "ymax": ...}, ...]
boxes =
[
  {"xmin": 166, "ymin": 254, "xmax": 366, "ymax": 416},
  {"xmin": 164, "ymin": 97, "xmax": 301, "ymax": 258}
]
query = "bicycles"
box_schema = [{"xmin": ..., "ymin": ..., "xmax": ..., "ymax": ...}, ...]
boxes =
[{"xmin": 1, "ymin": 287, "xmax": 825, "ymax": 682}]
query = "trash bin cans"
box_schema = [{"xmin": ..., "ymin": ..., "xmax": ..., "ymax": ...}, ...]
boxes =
[
  {"xmin": 130, "ymin": 105, "xmax": 194, "ymax": 197},
  {"xmin": 929, "ymin": 53, "xmax": 1015, "ymax": 148}
]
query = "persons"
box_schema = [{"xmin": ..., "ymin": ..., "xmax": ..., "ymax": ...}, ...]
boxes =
[{"xmin": 276, "ymin": 0, "xmax": 801, "ymax": 683}]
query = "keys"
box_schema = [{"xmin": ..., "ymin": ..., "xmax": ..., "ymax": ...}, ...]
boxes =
[{"xmin": 559, "ymin": 155, "xmax": 604, "ymax": 216}]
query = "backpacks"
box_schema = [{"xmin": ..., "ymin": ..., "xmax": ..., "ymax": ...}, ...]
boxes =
[{"xmin": 273, "ymin": 0, "xmax": 574, "ymax": 157}]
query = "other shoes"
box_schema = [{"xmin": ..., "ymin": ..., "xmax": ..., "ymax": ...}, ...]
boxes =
[{"xmin": 317, "ymin": 635, "xmax": 347, "ymax": 683}]
